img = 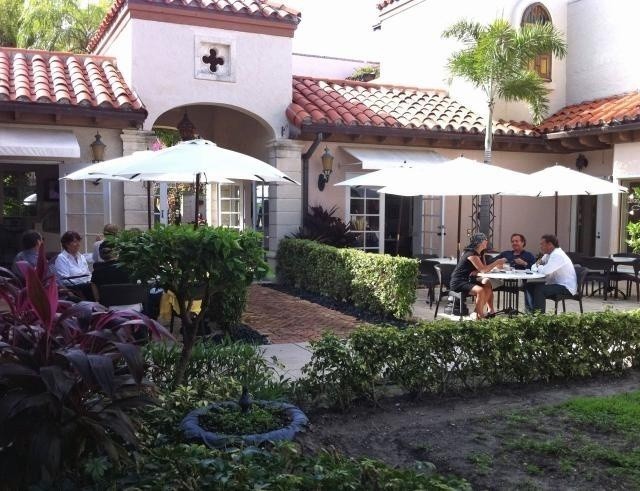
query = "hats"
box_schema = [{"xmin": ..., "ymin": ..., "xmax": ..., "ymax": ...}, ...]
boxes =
[
  {"xmin": 98, "ymin": 240, "xmax": 122, "ymax": 256},
  {"xmin": 465, "ymin": 231, "xmax": 489, "ymax": 248}
]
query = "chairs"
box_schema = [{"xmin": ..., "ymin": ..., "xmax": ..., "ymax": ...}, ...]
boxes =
[
  {"xmin": 415, "ymin": 252, "xmax": 640, "ymax": 321},
  {"xmin": 13, "ymin": 254, "xmax": 163, "ymax": 338}
]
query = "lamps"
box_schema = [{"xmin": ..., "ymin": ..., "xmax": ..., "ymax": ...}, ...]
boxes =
[
  {"xmin": 89, "ymin": 128, "xmax": 106, "ymax": 185},
  {"xmin": 576, "ymin": 154, "xmax": 587, "ymax": 170},
  {"xmin": 319, "ymin": 145, "xmax": 333, "ymax": 190}
]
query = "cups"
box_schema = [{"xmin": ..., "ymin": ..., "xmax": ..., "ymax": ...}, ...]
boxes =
[{"xmin": 491, "ymin": 262, "xmax": 543, "ymax": 275}]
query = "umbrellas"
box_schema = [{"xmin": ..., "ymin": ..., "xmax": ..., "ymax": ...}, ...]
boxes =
[
  {"xmin": 113, "ymin": 139, "xmax": 302, "ymax": 229},
  {"xmin": 57, "ymin": 147, "xmax": 235, "ymax": 229},
  {"xmin": 377, "ymin": 154, "xmax": 534, "ymax": 267},
  {"xmin": 332, "ymin": 160, "xmax": 432, "ymax": 257},
  {"xmin": 496, "ymin": 164, "xmax": 628, "ymax": 249}
]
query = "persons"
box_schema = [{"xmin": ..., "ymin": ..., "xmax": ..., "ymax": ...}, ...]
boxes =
[
  {"xmin": 525, "ymin": 233, "xmax": 578, "ymax": 315},
  {"xmin": 489, "ymin": 234, "xmax": 534, "ymax": 270},
  {"xmin": 450, "ymin": 232, "xmax": 507, "ymax": 321},
  {"xmin": 12, "ymin": 223, "xmax": 161, "ymax": 307}
]
